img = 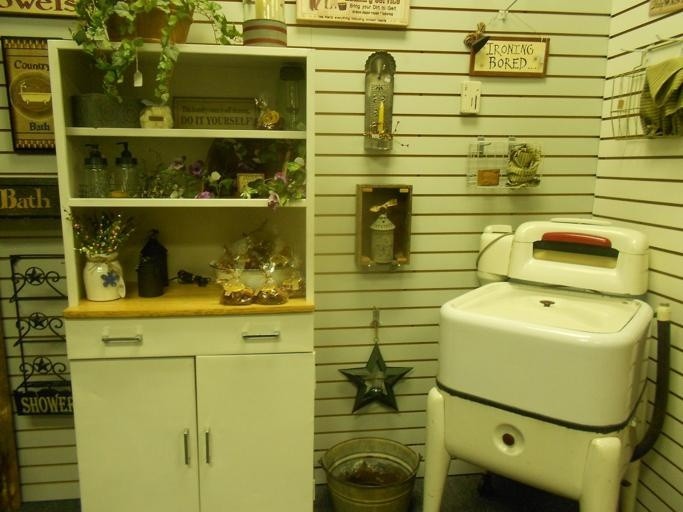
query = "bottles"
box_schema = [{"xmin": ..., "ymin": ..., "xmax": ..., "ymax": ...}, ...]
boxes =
[
  {"xmin": 370, "ymin": 214, "xmax": 396, "ymax": 262},
  {"xmin": 136, "ymin": 230, "xmax": 168, "ymax": 300}
]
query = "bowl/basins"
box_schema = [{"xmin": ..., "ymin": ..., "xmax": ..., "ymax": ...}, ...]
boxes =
[{"xmin": 206, "ymin": 257, "xmax": 292, "ymax": 293}]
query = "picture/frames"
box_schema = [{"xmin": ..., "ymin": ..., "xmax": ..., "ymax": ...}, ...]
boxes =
[
  {"xmin": 466, "ymin": 32, "xmax": 549, "ymax": 78},
  {"xmin": 0, "ymin": 33, "xmax": 55, "ymax": 155}
]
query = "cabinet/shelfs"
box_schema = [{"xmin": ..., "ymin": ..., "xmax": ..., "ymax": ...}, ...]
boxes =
[
  {"xmin": 61, "ymin": 295, "xmax": 319, "ymax": 510},
  {"xmin": 48, "ymin": 37, "xmax": 315, "ymax": 313}
]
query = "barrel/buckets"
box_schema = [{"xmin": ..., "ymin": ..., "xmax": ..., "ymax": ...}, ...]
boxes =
[{"xmin": 318, "ymin": 437, "xmax": 422, "ymax": 512}]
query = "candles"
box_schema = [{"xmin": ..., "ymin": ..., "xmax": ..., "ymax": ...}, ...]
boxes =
[{"xmin": 376, "ymin": 100, "xmax": 384, "ymax": 135}]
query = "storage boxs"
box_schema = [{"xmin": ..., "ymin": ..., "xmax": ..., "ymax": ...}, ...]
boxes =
[{"xmin": 71, "ymin": 90, "xmax": 145, "ymax": 128}]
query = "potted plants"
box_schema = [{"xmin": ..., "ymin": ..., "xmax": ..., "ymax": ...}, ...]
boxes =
[{"xmin": 71, "ymin": 0, "xmax": 244, "ymax": 109}]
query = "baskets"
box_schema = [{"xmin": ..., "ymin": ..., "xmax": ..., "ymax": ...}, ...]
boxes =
[
  {"xmin": 467, "ymin": 140, "xmax": 543, "ymax": 190},
  {"xmin": 611, "ymin": 68, "xmax": 683, "ymax": 139}
]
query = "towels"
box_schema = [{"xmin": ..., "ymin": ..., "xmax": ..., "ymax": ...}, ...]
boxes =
[
  {"xmin": 503, "ymin": 142, "xmax": 542, "ymax": 190},
  {"xmin": 639, "ymin": 55, "xmax": 682, "ymax": 139}
]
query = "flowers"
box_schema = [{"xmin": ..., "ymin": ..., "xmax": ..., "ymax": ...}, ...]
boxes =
[
  {"xmin": 171, "ymin": 136, "xmax": 306, "ymax": 214},
  {"xmin": 60, "ymin": 200, "xmax": 137, "ymax": 257}
]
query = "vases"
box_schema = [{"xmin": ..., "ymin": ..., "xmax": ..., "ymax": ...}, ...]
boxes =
[{"xmin": 83, "ymin": 251, "xmax": 124, "ymax": 302}]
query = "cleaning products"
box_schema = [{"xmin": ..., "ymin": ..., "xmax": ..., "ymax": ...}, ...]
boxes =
[
  {"xmin": 79, "ymin": 143, "xmax": 111, "ymax": 198},
  {"xmin": 110, "ymin": 142, "xmax": 139, "ymax": 197}
]
